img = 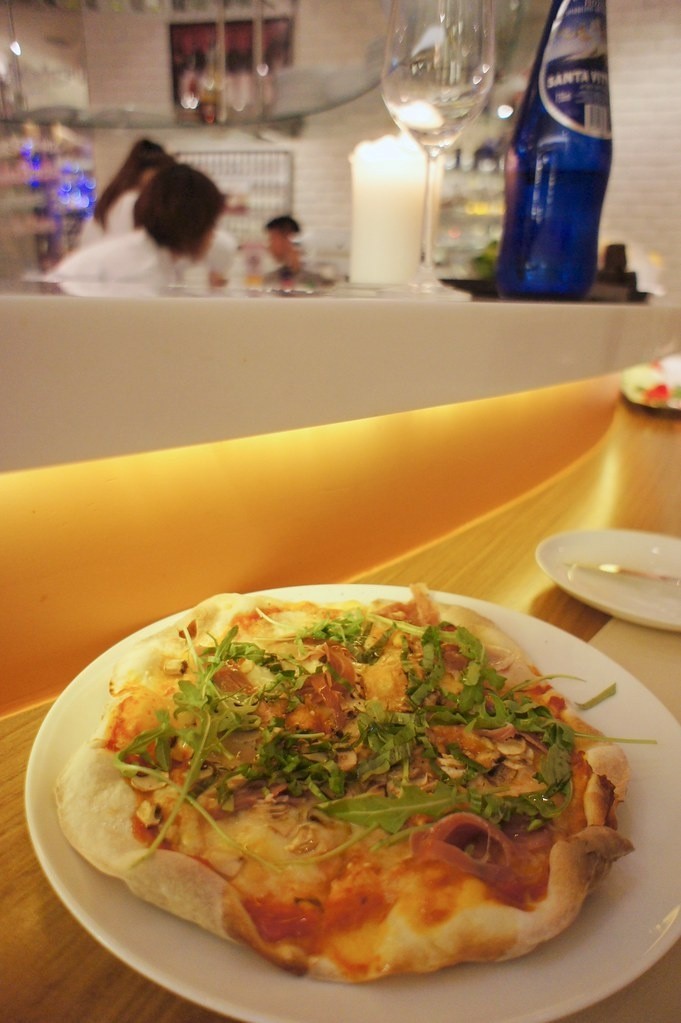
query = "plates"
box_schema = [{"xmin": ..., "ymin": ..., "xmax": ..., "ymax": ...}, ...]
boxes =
[
  {"xmin": 619, "ymin": 364, "xmax": 681, "ymax": 413},
  {"xmin": 22, "ymin": 583, "xmax": 681, "ymax": 1023},
  {"xmin": 536, "ymin": 528, "xmax": 681, "ymax": 632}
]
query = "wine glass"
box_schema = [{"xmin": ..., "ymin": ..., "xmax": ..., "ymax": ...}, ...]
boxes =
[{"xmin": 377, "ymin": 0, "xmax": 495, "ymax": 303}]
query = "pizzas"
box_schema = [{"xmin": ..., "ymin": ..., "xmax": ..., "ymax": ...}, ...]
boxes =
[{"xmin": 53, "ymin": 581, "xmax": 638, "ymax": 983}]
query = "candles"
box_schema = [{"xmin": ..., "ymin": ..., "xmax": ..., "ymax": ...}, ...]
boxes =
[{"xmin": 347, "ymin": 131, "xmax": 431, "ymax": 287}]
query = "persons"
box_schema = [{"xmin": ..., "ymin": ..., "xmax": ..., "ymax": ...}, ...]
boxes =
[
  {"xmin": 255, "ymin": 217, "xmax": 334, "ymax": 288},
  {"xmin": 46, "ymin": 135, "xmax": 230, "ymax": 294}
]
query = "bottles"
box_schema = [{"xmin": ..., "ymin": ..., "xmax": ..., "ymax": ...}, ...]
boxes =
[{"xmin": 492, "ymin": 0, "xmax": 613, "ymax": 300}]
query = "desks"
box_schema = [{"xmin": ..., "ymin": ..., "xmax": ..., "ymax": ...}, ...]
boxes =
[{"xmin": 0, "ymin": 370, "xmax": 681, "ymax": 1023}]
